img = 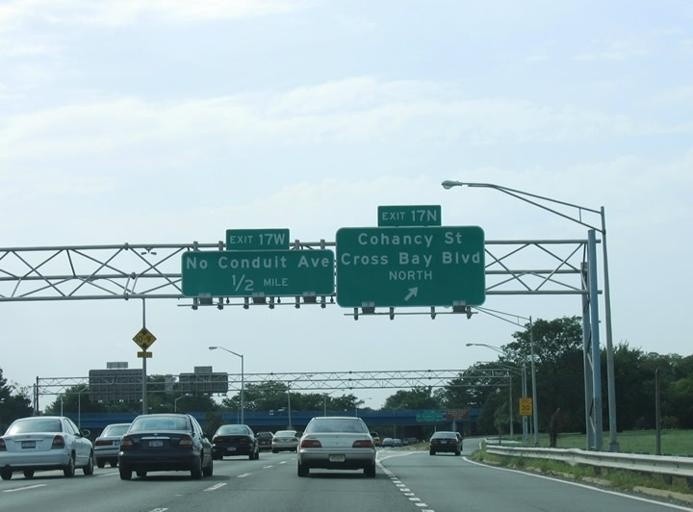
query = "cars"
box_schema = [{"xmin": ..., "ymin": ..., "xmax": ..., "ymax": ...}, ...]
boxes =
[
  {"xmin": 253, "ymin": 431, "xmax": 275, "ymax": 452},
  {"xmin": 210, "ymin": 424, "xmax": 258, "ymax": 461},
  {"xmin": 117, "ymin": 414, "xmax": 213, "ymax": 480},
  {"xmin": 295, "ymin": 416, "xmax": 377, "ymax": 477},
  {"xmin": 429, "ymin": 429, "xmax": 463, "ymax": 455},
  {"xmin": 271, "ymin": 430, "xmax": 302, "ymax": 453},
  {"xmin": 92, "ymin": 421, "xmax": 132, "ymax": 467},
  {"xmin": 0, "ymin": 416, "xmax": 94, "ymax": 479},
  {"xmin": 381, "ymin": 436, "xmax": 419, "ymax": 449}
]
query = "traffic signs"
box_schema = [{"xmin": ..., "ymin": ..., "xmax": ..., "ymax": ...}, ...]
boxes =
[
  {"xmin": 416, "ymin": 409, "xmax": 447, "ymax": 423},
  {"xmin": 181, "ymin": 249, "xmax": 334, "ymax": 297},
  {"xmin": 519, "ymin": 398, "xmax": 532, "ymax": 416},
  {"xmin": 226, "ymin": 228, "xmax": 288, "ymax": 250},
  {"xmin": 336, "ymin": 226, "xmax": 485, "ymax": 307},
  {"xmin": 377, "ymin": 205, "xmax": 440, "ymax": 226}
]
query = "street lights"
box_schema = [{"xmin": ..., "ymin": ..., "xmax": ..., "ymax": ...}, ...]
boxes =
[
  {"xmin": 322, "ymin": 388, "xmax": 343, "ymax": 417},
  {"xmin": 465, "ymin": 341, "xmax": 530, "ymax": 441},
  {"xmin": 440, "ymin": 179, "xmax": 619, "ymax": 455},
  {"xmin": 207, "ymin": 345, "xmax": 245, "ymax": 423},
  {"xmin": 222, "ymin": 394, "xmax": 239, "ymax": 422},
  {"xmin": 173, "ymin": 392, "xmax": 190, "ymax": 415},
  {"xmin": 77, "ymin": 385, "xmax": 94, "ymax": 430},
  {"xmin": 40, "ymin": 388, "xmax": 63, "ymax": 416},
  {"xmin": 353, "ymin": 396, "xmax": 372, "ymax": 417},
  {"xmin": 468, "ymin": 366, "xmax": 514, "ymax": 439},
  {"xmin": 286, "ymin": 373, "xmax": 317, "ymax": 429}
]
